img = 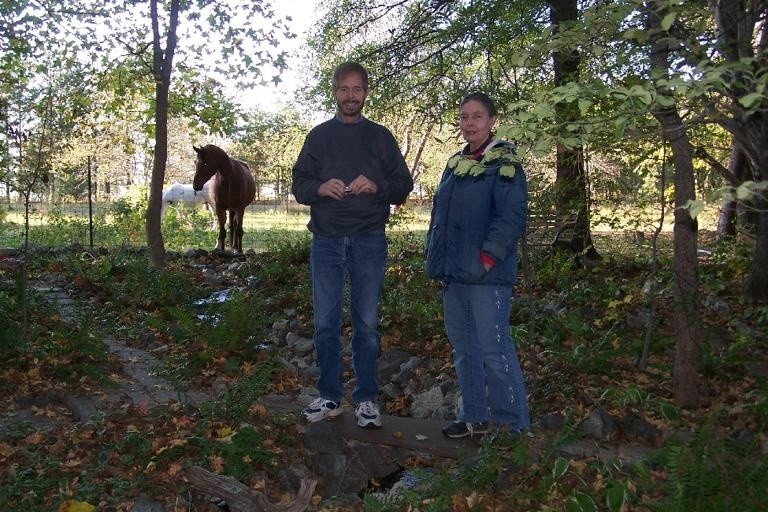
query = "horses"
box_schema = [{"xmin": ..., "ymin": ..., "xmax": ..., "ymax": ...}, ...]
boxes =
[
  {"xmin": 191, "ymin": 143, "xmax": 256, "ymax": 253},
  {"xmin": 159, "ymin": 180, "xmax": 218, "ymax": 232}
]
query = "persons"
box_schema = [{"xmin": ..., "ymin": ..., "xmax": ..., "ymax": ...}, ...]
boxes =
[
  {"xmin": 424, "ymin": 91, "xmax": 531, "ymax": 438},
  {"xmin": 291, "ymin": 63, "xmax": 414, "ymax": 429}
]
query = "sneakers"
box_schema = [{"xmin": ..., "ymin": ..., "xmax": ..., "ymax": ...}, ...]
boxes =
[
  {"xmin": 442, "ymin": 421, "xmax": 521, "ymax": 449},
  {"xmin": 355, "ymin": 401, "xmax": 382, "ymax": 428},
  {"xmin": 301, "ymin": 398, "xmax": 342, "ymax": 423}
]
